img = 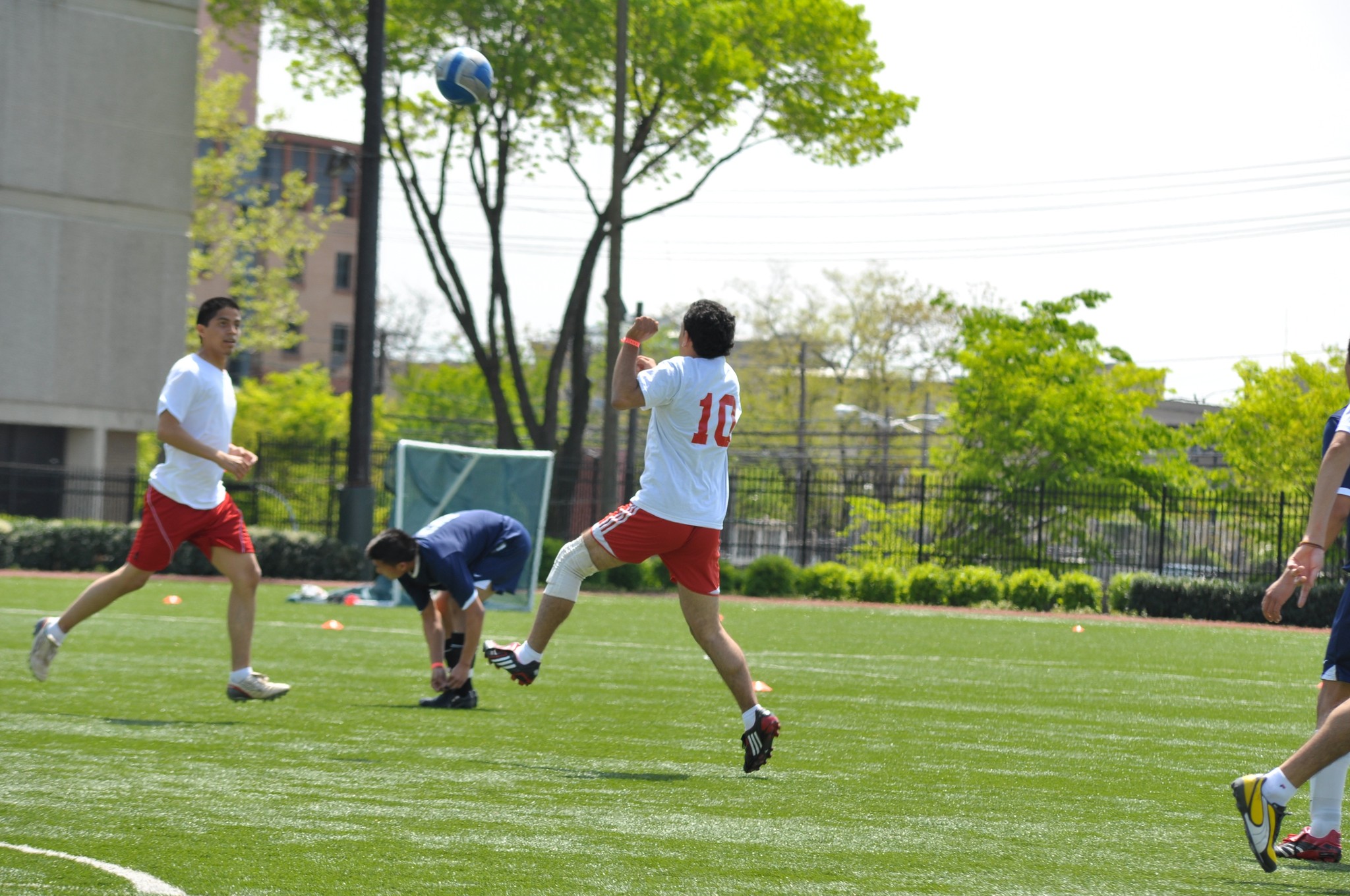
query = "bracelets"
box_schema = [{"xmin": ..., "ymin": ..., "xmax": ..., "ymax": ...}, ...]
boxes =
[
  {"xmin": 621, "ymin": 337, "xmax": 639, "ymax": 347},
  {"xmin": 1298, "ymin": 541, "xmax": 1325, "ymax": 551},
  {"xmin": 430, "ymin": 662, "xmax": 443, "ymax": 668}
]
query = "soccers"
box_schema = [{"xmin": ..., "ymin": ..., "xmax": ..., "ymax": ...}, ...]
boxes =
[{"xmin": 436, "ymin": 47, "xmax": 494, "ymax": 106}]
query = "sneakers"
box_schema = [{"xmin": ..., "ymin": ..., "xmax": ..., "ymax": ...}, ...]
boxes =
[
  {"xmin": 484, "ymin": 640, "xmax": 540, "ymax": 686},
  {"xmin": 1274, "ymin": 826, "xmax": 1342, "ymax": 863},
  {"xmin": 1231, "ymin": 772, "xmax": 1292, "ymax": 873},
  {"xmin": 420, "ymin": 685, "xmax": 478, "ymax": 710},
  {"xmin": 227, "ymin": 671, "xmax": 291, "ymax": 704},
  {"xmin": 27, "ymin": 617, "xmax": 59, "ymax": 682},
  {"xmin": 741, "ymin": 707, "xmax": 780, "ymax": 773}
]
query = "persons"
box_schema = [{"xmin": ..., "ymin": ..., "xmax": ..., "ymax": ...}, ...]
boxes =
[
  {"xmin": 483, "ymin": 300, "xmax": 780, "ymax": 773},
  {"xmin": 1231, "ymin": 339, "xmax": 1350, "ymax": 871},
  {"xmin": 26, "ymin": 296, "xmax": 292, "ymax": 703},
  {"xmin": 364, "ymin": 510, "xmax": 533, "ymax": 709}
]
